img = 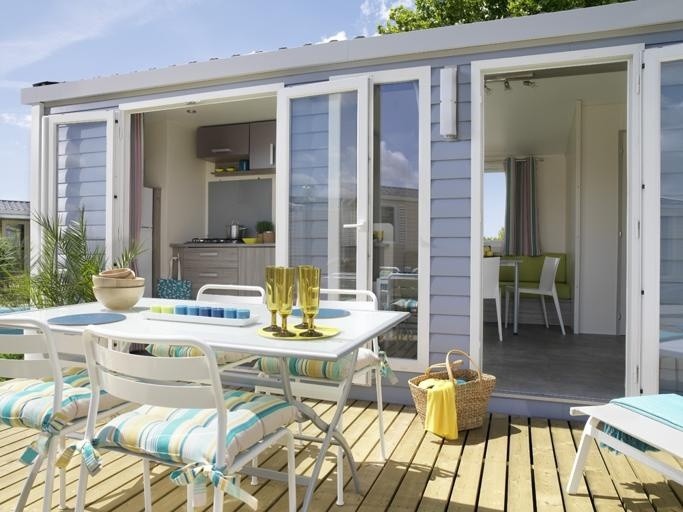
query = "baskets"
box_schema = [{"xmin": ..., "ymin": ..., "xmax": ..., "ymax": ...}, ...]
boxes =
[{"xmin": 407, "ymin": 348, "xmax": 496, "ymax": 433}]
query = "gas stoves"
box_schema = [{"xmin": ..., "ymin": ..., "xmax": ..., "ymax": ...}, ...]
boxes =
[{"xmin": 185, "ymin": 237, "xmax": 231, "ymax": 244}]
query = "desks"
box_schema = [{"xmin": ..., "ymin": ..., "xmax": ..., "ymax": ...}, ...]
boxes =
[{"xmin": 5, "ymin": 302, "xmax": 411, "ymax": 512}]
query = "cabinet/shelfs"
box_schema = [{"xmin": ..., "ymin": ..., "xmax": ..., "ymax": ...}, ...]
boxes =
[
  {"xmin": 238, "ymin": 247, "xmax": 275, "ymax": 296},
  {"xmin": 194, "ymin": 123, "xmax": 249, "ymax": 164},
  {"xmin": 172, "ymin": 248, "xmax": 238, "ymax": 301},
  {"xmin": 249, "ymin": 120, "xmax": 275, "ymax": 169}
]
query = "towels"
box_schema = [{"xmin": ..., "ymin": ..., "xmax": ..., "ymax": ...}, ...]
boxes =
[{"xmin": 424, "ymin": 378, "xmax": 460, "ymax": 440}]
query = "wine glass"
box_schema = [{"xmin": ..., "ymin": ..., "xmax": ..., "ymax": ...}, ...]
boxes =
[{"xmin": 262, "ymin": 265, "xmax": 323, "ymax": 337}]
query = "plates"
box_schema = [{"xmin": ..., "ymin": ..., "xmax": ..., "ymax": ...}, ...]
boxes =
[
  {"xmin": 213, "ymin": 168, "xmax": 237, "ymax": 172},
  {"xmin": 255, "ymin": 323, "xmax": 342, "ymax": 341}
]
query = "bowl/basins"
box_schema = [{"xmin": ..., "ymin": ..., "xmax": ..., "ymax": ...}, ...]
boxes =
[
  {"xmin": 91, "ymin": 267, "xmax": 145, "ymax": 311},
  {"xmin": 240, "ymin": 236, "xmax": 257, "ymax": 244}
]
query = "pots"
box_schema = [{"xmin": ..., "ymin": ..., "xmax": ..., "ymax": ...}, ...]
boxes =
[{"xmin": 225, "ymin": 224, "xmax": 248, "ymax": 239}]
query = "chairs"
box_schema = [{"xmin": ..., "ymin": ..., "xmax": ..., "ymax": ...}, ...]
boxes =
[
  {"xmin": 2, "ymin": 284, "xmax": 399, "ymax": 508},
  {"xmin": 377, "ymin": 265, "xmax": 418, "ymax": 354},
  {"xmin": 482, "ymin": 253, "xmax": 568, "ymax": 342}
]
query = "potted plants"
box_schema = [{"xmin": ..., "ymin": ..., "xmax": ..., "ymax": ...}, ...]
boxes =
[
  {"xmin": 0, "ymin": 209, "xmax": 149, "ymax": 362},
  {"xmin": 254, "ymin": 221, "xmax": 273, "ymax": 244}
]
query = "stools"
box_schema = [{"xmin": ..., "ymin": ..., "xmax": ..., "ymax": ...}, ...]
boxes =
[{"xmin": 562, "ymin": 393, "xmax": 683, "ymax": 499}]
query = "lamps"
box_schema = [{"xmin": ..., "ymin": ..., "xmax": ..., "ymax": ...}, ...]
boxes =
[{"xmin": 482, "ymin": 76, "xmax": 538, "ymax": 96}]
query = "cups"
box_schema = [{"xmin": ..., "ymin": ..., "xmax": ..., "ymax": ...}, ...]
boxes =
[
  {"xmin": 223, "ymin": 307, "xmax": 235, "ymax": 319},
  {"xmin": 186, "ymin": 304, "xmax": 198, "ymax": 315},
  {"xmin": 160, "ymin": 304, "xmax": 174, "ymax": 314},
  {"xmin": 149, "ymin": 304, "xmax": 162, "ymax": 314},
  {"xmin": 210, "ymin": 306, "xmax": 223, "ymax": 317},
  {"xmin": 239, "ymin": 159, "xmax": 249, "ymax": 171},
  {"xmin": 198, "ymin": 305, "xmax": 210, "ymax": 316},
  {"xmin": 234, "ymin": 308, "xmax": 250, "ymax": 319},
  {"xmin": 174, "ymin": 304, "xmax": 186, "ymax": 314}
]
